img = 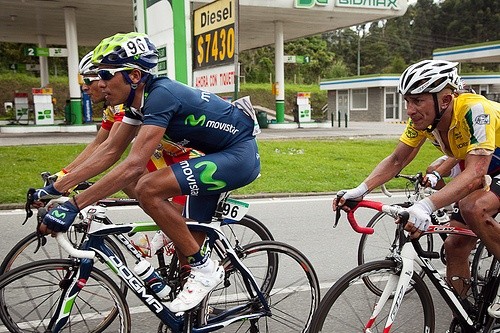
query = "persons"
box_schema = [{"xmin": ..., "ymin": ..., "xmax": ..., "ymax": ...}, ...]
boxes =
[
  {"xmin": 334, "ymin": 60, "xmax": 500, "ymax": 333},
  {"xmin": 420, "ymin": 81, "xmax": 477, "ymax": 297},
  {"xmin": 38, "ymin": 33, "xmax": 260, "ymax": 318},
  {"xmin": 48, "ymin": 50, "xmax": 205, "ymax": 255}
]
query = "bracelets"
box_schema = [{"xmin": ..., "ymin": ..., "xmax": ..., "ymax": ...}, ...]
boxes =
[
  {"xmin": 73, "ymin": 194, "xmax": 79, "ymax": 210},
  {"xmin": 433, "ymin": 172, "xmax": 440, "ymax": 179}
]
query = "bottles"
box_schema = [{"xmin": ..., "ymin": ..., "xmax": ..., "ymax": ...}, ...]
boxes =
[
  {"xmin": 133, "ymin": 259, "xmax": 172, "ymax": 298},
  {"xmin": 436, "ymin": 210, "xmax": 451, "ymax": 237},
  {"xmin": 128, "ymin": 231, "xmax": 156, "ymax": 259}
]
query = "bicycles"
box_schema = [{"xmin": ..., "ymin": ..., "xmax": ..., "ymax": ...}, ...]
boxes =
[
  {"xmin": 0, "ymin": 171, "xmax": 280, "ymax": 333},
  {"xmin": 357, "ymin": 171, "xmax": 500, "ymax": 299},
  {"xmin": 307, "ymin": 190, "xmax": 500, "ymax": 333},
  {"xmin": 0, "ymin": 173, "xmax": 321, "ymax": 333}
]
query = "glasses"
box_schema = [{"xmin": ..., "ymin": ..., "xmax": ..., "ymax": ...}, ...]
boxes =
[
  {"xmin": 82, "ymin": 76, "xmax": 101, "ymax": 85},
  {"xmin": 97, "ymin": 67, "xmax": 136, "ymax": 81}
]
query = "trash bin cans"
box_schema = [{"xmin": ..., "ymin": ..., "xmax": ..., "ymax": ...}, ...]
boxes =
[
  {"xmin": 257, "ymin": 115, "xmax": 262, "ymax": 128},
  {"xmin": 64, "ymin": 105, "xmax": 70, "ymax": 122},
  {"xmin": 261, "ymin": 113, "xmax": 267, "ymax": 128},
  {"xmin": 95, "ymin": 123, "xmax": 102, "ymax": 131}
]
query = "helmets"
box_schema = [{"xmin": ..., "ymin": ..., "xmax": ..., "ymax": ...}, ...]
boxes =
[
  {"xmin": 79, "ymin": 50, "xmax": 102, "ymax": 75},
  {"xmin": 399, "ymin": 59, "xmax": 462, "ymax": 95},
  {"xmin": 91, "ymin": 31, "xmax": 159, "ymax": 75}
]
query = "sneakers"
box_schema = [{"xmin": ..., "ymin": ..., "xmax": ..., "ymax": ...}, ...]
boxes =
[
  {"xmin": 150, "ymin": 228, "xmax": 172, "ymax": 249},
  {"xmin": 169, "ymin": 259, "xmax": 225, "ymax": 313},
  {"xmin": 488, "ymin": 286, "xmax": 500, "ymax": 319}
]
